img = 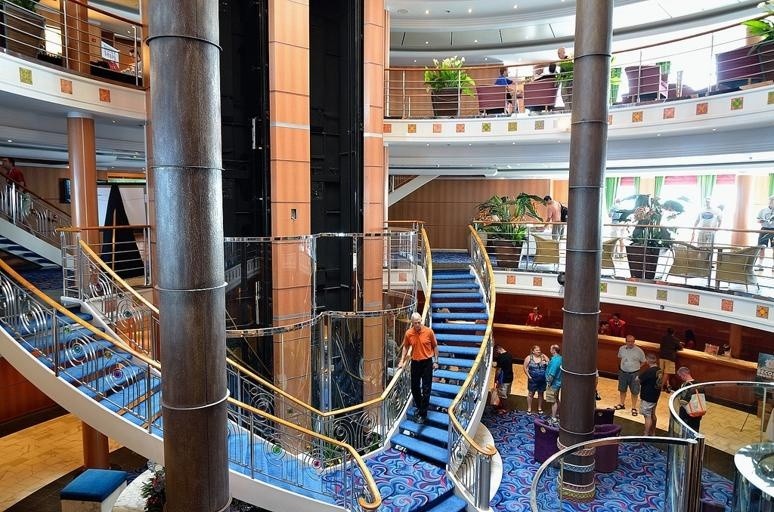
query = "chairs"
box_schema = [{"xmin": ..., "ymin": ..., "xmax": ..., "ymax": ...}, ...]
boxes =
[
  {"xmin": 476, "ymin": 74, "xmax": 561, "ymax": 116},
  {"xmin": 624, "ymin": 64, "xmax": 669, "ymax": 100},
  {"xmin": 534, "ymin": 418, "xmax": 622, "ymax": 473},
  {"xmin": 530, "ymin": 232, "xmax": 621, "ymax": 274},
  {"xmin": 665, "ymin": 239, "xmax": 762, "ymax": 293}
]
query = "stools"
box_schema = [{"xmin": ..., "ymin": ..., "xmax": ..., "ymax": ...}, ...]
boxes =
[{"xmin": 59, "ymin": 469, "xmax": 128, "ymax": 512}]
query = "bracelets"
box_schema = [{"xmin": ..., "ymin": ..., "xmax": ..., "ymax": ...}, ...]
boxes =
[{"xmin": 434, "ymin": 362, "xmax": 438, "ymax": 364}]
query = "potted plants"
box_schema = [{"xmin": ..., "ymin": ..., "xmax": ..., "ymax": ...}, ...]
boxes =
[
  {"xmin": 464, "ymin": 190, "xmax": 548, "ymax": 268},
  {"xmin": 610, "ymin": 193, "xmax": 684, "ymax": 278},
  {"xmin": 424, "ymin": 55, "xmax": 476, "ymax": 116}
]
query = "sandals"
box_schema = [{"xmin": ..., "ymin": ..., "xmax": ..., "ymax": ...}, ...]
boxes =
[
  {"xmin": 537, "ymin": 409, "xmax": 543, "ymax": 414},
  {"xmin": 613, "ymin": 404, "xmax": 625, "ymax": 410},
  {"xmin": 632, "ymin": 408, "xmax": 637, "ymax": 416},
  {"xmin": 526, "ymin": 409, "xmax": 531, "ymax": 416},
  {"xmin": 496, "ymin": 408, "xmax": 509, "ymax": 416}
]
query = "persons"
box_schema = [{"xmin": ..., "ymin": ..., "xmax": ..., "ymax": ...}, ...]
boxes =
[
  {"xmin": 492, "ymin": 342, "xmax": 513, "ymax": 414},
  {"xmin": 596, "ymin": 321, "xmax": 610, "ymax": 336},
  {"xmin": 612, "ymin": 335, "xmax": 645, "ymax": 416},
  {"xmin": 683, "ymin": 330, "xmax": 697, "ymax": 350},
  {"xmin": 755, "ymin": 195, "xmax": 774, "ymax": 272},
  {"xmin": 541, "ymin": 194, "xmax": 567, "ymax": 242},
  {"xmin": 635, "ymin": 353, "xmax": 663, "ymax": 436},
  {"xmin": 718, "ymin": 342, "xmax": 732, "ymax": 357},
  {"xmin": 691, "ymin": 195, "xmax": 723, "ymax": 267},
  {"xmin": 558, "ymin": 47, "xmax": 569, "ymax": 60},
  {"xmin": 495, "ymin": 66, "xmax": 517, "ymax": 114},
  {"xmin": 125, "ymin": 47, "xmax": 142, "ymax": 78},
  {"xmin": 398, "ymin": 311, "xmax": 439, "ymax": 426},
  {"xmin": 674, "ymin": 366, "xmax": 702, "ymax": 434},
  {"xmin": 592, "ymin": 367, "xmax": 602, "ymax": 401},
  {"xmin": 535, "ymin": 63, "xmax": 559, "ymax": 80},
  {"xmin": 658, "ymin": 326, "xmax": 683, "ymax": 393},
  {"xmin": 545, "ymin": 344, "xmax": 563, "ymax": 425},
  {"xmin": 608, "ymin": 197, "xmax": 630, "ymax": 260},
  {"xmin": 522, "ymin": 346, "xmax": 550, "ymax": 417},
  {"xmin": 526, "ymin": 305, "xmax": 546, "ymax": 327},
  {"xmin": 610, "ymin": 313, "xmax": 627, "ymax": 338}
]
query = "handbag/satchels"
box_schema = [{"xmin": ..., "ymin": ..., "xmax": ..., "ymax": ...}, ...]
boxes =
[
  {"xmin": 680, "ymin": 393, "xmax": 706, "ymax": 418},
  {"xmin": 561, "ymin": 206, "xmax": 568, "ymax": 222}
]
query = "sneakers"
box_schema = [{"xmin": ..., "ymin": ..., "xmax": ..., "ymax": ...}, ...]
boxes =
[
  {"xmin": 414, "ymin": 408, "xmax": 424, "ymax": 423},
  {"xmin": 667, "ymin": 384, "xmax": 671, "ymax": 392},
  {"xmin": 546, "ymin": 417, "xmax": 559, "ymax": 425},
  {"xmin": 595, "ymin": 390, "xmax": 600, "ymax": 399}
]
